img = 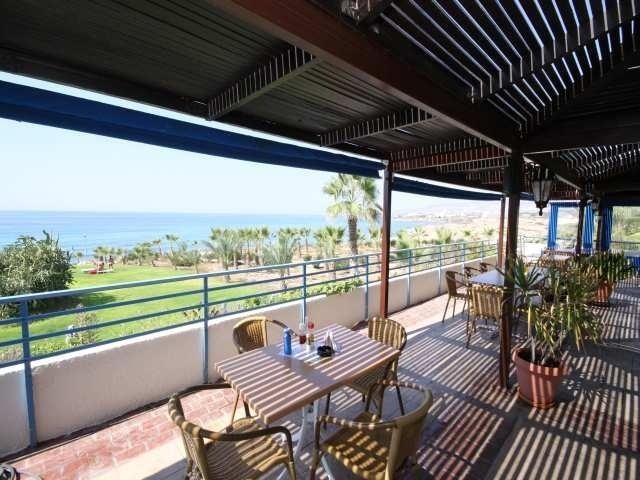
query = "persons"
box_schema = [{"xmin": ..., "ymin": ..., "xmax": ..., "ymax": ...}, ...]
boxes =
[{"xmin": 109, "ymin": 256, "xmax": 113, "ymax": 269}]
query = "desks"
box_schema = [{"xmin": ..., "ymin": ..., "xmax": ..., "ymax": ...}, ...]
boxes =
[{"xmin": 214, "ymin": 321, "xmax": 401, "ymax": 436}]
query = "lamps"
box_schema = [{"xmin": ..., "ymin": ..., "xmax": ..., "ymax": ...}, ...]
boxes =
[
  {"xmin": 524, "ymin": 157, "xmax": 560, "ymax": 217},
  {"xmin": 584, "ymin": 194, "xmax": 600, "ymax": 215}
]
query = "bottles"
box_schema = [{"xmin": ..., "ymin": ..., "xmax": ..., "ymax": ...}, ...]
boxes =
[
  {"xmin": 283, "ymin": 327, "xmax": 292, "ymax": 356},
  {"xmin": 543, "ymin": 246, "xmax": 546, "ymax": 254},
  {"xmin": 306, "ymin": 321, "xmax": 315, "ymax": 348},
  {"xmin": 299, "ymin": 323, "xmax": 307, "ymax": 344},
  {"xmin": 524, "ymin": 256, "xmax": 529, "ymax": 272}
]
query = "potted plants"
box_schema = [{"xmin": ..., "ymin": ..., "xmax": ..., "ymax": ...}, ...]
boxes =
[
  {"xmin": 500, "ymin": 256, "xmax": 604, "ymax": 411},
  {"xmin": 567, "ymin": 251, "xmax": 639, "ymax": 306}
]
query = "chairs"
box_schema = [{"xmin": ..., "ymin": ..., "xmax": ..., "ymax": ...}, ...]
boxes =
[
  {"xmin": 165, "ymin": 381, "xmax": 299, "ymax": 480},
  {"xmin": 323, "ymin": 318, "xmax": 409, "ymax": 423},
  {"xmin": 445, "ymin": 259, "xmax": 558, "ymax": 346},
  {"xmin": 308, "ymin": 380, "xmax": 435, "ymax": 480},
  {"xmin": 228, "ymin": 314, "xmax": 298, "ymax": 426},
  {"xmin": 533, "ymin": 248, "xmax": 576, "ymax": 268}
]
